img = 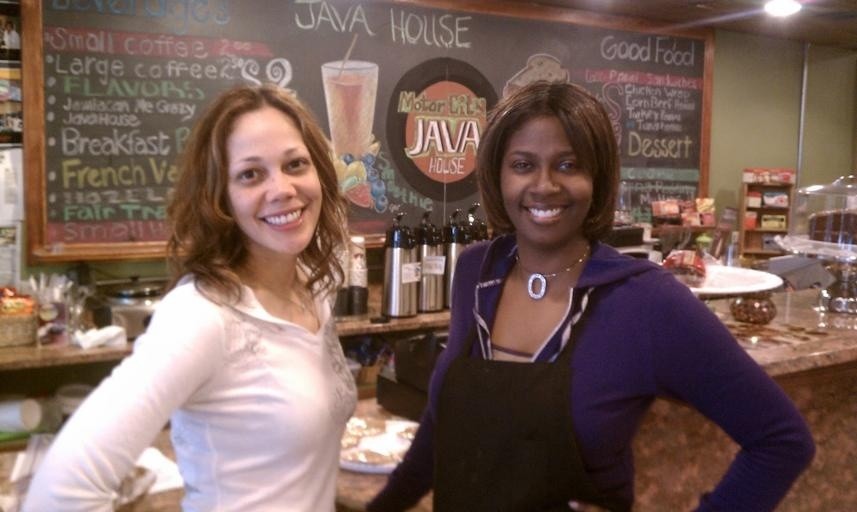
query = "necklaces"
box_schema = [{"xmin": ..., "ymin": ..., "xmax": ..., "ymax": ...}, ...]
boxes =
[{"xmin": 510, "ymin": 244, "xmax": 591, "ymax": 301}]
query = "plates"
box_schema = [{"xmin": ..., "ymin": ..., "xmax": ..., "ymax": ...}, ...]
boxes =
[
  {"xmin": 56, "ymin": 382, "xmax": 101, "ymax": 415},
  {"xmin": 691, "ymin": 266, "xmax": 784, "ymax": 296}
]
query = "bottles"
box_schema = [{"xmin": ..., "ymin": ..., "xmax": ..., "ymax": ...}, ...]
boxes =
[
  {"xmin": 725, "ymin": 232, "xmax": 741, "ymax": 268},
  {"xmin": 707, "ymin": 208, "xmax": 739, "ymax": 257}
]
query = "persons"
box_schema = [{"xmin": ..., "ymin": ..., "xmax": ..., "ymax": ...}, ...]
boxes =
[
  {"xmin": 345, "ymin": 78, "xmax": 817, "ymax": 512},
  {"xmin": 25, "ymin": 84, "xmax": 361, "ymax": 512}
]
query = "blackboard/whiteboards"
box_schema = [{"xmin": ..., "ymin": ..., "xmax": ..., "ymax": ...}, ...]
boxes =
[{"xmin": 18, "ymin": 0, "xmax": 717, "ymax": 268}]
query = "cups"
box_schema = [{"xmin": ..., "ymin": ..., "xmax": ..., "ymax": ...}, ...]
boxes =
[
  {"xmin": 36, "ymin": 302, "xmax": 67, "ymax": 346},
  {"xmin": 332, "ymin": 235, "xmax": 370, "ymax": 314},
  {"xmin": 318, "ymin": 60, "xmax": 379, "ymax": 157}
]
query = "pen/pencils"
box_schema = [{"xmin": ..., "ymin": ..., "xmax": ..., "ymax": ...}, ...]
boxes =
[{"xmin": 28, "ymin": 272, "xmax": 76, "ymax": 303}]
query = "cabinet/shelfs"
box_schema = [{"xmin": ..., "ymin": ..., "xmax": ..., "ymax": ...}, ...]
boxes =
[{"xmin": 738, "ymin": 169, "xmax": 795, "ymax": 260}]
